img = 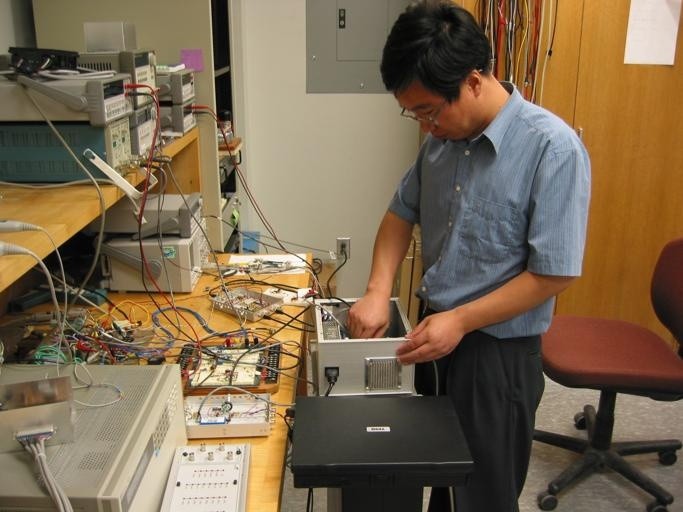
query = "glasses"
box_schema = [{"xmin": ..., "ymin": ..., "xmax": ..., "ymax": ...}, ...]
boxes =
[{"xmin": 400, "ymin": 101, "xmax": 445, "ymax": 123}]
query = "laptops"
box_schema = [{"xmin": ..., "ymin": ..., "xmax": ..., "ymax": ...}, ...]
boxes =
[{"xmin": 291, "ymin": 395, "xmax": 475, "ymax": 474}]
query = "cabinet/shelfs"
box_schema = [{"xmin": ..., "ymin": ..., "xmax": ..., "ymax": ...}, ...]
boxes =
[{"xmin": 31, "ymin": 0, "xmax": 240, "ymax": 253}]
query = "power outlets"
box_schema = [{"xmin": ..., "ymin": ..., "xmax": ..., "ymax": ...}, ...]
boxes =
[{"xmin": 337, "ymin": 238, "xmax": 349, "ymax": 259}]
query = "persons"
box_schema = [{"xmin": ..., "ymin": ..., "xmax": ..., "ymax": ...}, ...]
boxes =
[{"xmin": 346, "ymin": 1, "xmax": 593, "ymax": 512}]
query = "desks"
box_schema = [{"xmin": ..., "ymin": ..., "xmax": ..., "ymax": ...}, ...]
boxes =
[{"xmin": 2, "ymin": 131, "xmax": 313, "ymax": 512}]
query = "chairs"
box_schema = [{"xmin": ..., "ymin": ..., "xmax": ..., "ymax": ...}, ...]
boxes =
[{"xmin": 533, "ymin": 238, "xmax": 683, "ymax": 512}]
